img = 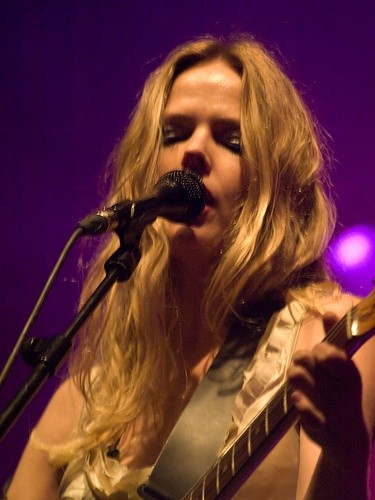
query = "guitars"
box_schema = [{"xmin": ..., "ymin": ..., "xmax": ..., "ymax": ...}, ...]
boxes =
[{"xmin": 174, "ymin": 286, "xmax": 375, "ymax": 500}]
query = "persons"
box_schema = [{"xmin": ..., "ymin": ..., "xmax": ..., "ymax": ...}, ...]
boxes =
[{"xmin": 1, "ymin": 32, "xmax": 375, "ymax": 499}]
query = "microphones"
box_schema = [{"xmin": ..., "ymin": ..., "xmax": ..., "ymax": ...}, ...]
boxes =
[{"xmin": 79, "ymin": 172, "xmax": 206, "ymax": 237}]
